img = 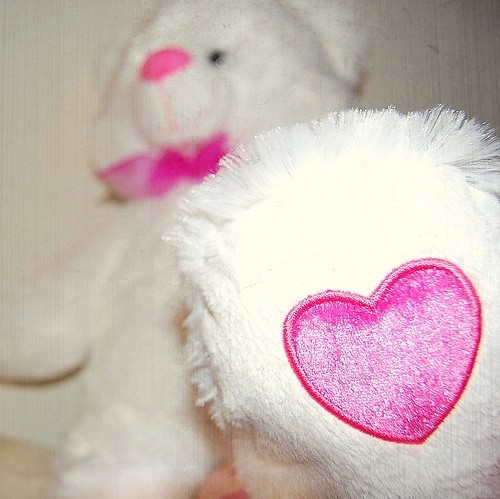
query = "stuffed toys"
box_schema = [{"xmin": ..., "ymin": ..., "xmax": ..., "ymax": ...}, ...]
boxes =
[{"xmin": 1, "ymin": 1, "xmax": 500, "ymax": 498}]
